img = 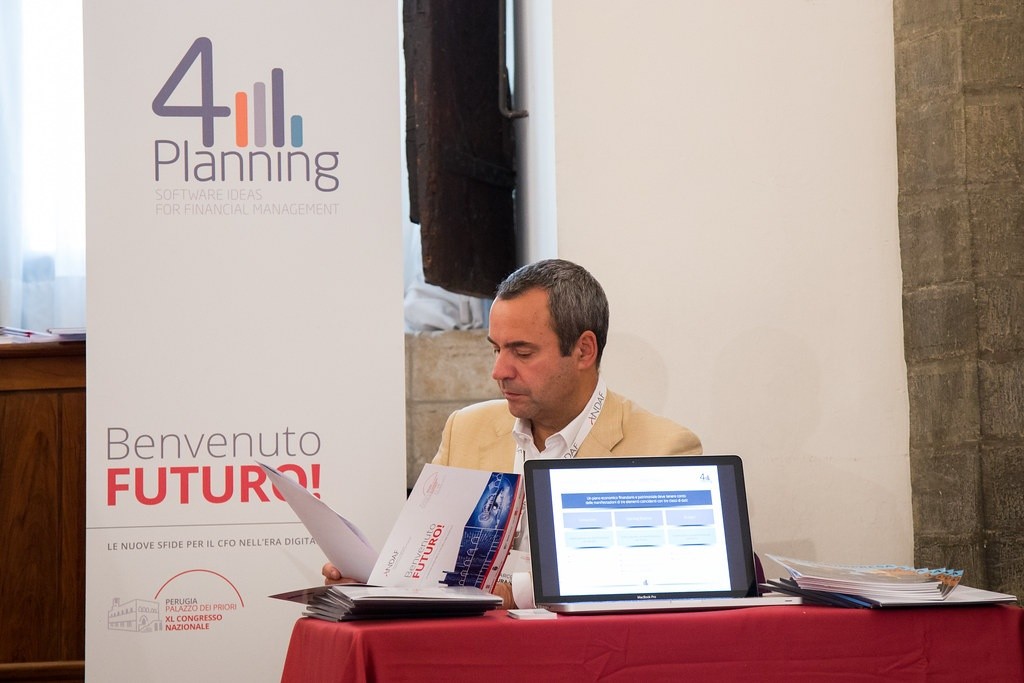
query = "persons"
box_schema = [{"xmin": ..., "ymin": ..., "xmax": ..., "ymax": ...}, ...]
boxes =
[{"xmin": 320, "ymin": 260, "xmax": 703, "ymax": 611}]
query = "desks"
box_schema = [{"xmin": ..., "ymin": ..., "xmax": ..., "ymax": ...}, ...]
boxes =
[
  {"xmin": 280, "ymin": 602, "xmax": 1024, "ymax": 683},
  {"xmin": 0, "ymin": 331, "xmax": 86, "ymax": 683}
]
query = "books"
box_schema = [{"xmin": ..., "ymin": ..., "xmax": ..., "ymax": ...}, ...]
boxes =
[
  {"xmin": 506, "ymin": 609, "xmax": 558, "ymax": 619},
  {"xmin": 301, "ymin": 586, "xmax": 504, "ymax": 623},
  {"xmin": 758, "ymin": 552, "xmax": 1018, "ymax": 609}
]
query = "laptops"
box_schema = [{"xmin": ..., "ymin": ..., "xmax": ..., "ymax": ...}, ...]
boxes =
[{"xmin": 524, "ymin": 455, "xmax": 804, "ymax": 613}]
